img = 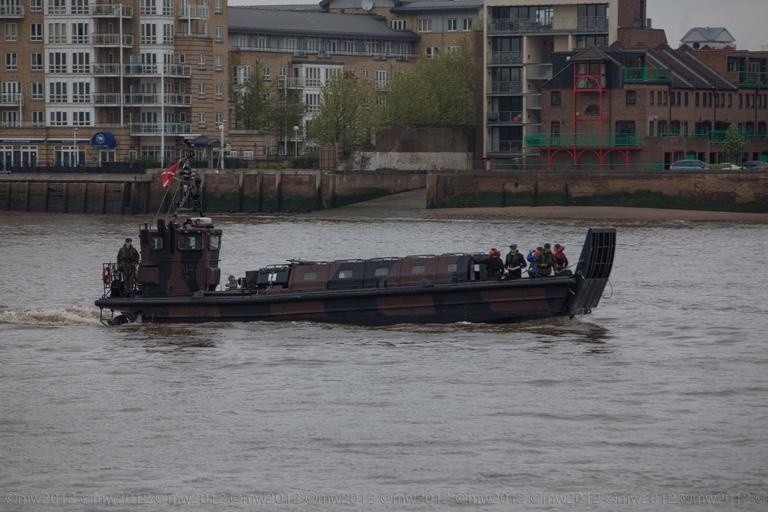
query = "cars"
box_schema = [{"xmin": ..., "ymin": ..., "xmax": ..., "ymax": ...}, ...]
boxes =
[{"xmin": 670, "ymin": 159, "xmax": 766, "ymax": 171}]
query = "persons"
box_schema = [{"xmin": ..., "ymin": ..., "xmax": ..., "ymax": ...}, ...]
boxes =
[
  {"xmin": 117, "ymin": 239, "xmax": 139, "ymax": 296},
  {"xmin": 487, "ymin": 243, "xmax": 567, "ymax": 281}
]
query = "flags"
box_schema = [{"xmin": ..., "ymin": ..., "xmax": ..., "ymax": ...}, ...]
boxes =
[{"xmin": 160, "ymin": 158, "xmax": 179, "ymax": 188}]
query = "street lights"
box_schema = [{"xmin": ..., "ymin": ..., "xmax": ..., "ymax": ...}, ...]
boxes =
[
  {"xmin": 215, "ymin": 120, "xmax": 227, "ymax": 169},
  {"xmin": 293, "ymin": 126, "xmax": 299, "ymax": 158}
]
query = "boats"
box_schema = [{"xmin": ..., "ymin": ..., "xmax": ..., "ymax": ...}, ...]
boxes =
[{"xmin": 95, "ymin": 217, "xmax": 617, "ymax": 328}]
